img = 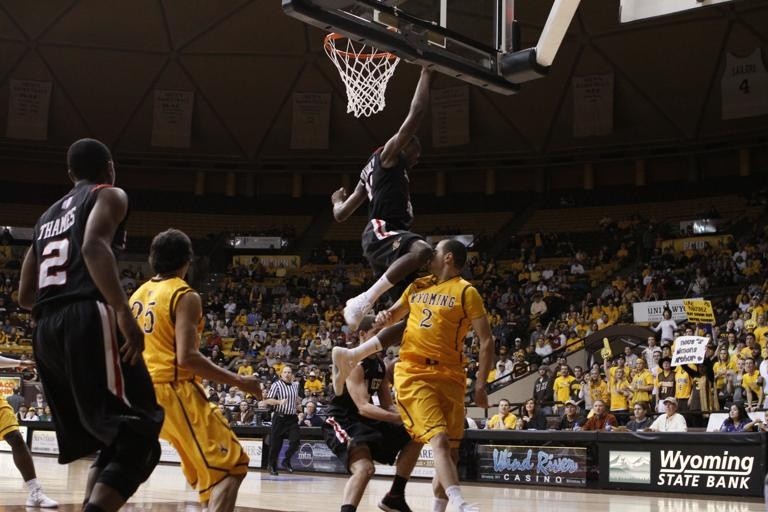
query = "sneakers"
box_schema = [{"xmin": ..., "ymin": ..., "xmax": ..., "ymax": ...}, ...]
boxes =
[
  {"xmin": 331, "ymin": 346, "xmax": 355, "ymax": 397},
  {"xmin": 341, "ymin": 291, "xmax": 372, "ymax": 331},
  {"xmin": 378, "ymin": 490, "xmax": 413, "ymax": 512},
  {"xmin": 444, "ymin": 497, "xmax": 481, "ymax": 512},
  {"xmin": 25, "ymin": 487, "xmax": 60, "ymax": 509}
]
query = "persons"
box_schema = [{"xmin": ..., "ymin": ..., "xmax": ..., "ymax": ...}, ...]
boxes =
[
  {"xmin": 299, "ymin": 401, "xmax": 322, "ymax": 427},
  {"xmin": 650, "ymin": 351, "xmax": 663, "ymax": 414},
  {"xmin": 496, "ymin": 348, "xmax": 513, "ymax": 371},
  {"xmin": 14, "ymin": 406, "xmax": 27, "ymax": 422},
  {"xmin": 496, "ymin": 362, "xmax": 511, "ymax": 386},
  {"xmin": 25, "ymin": 406, "xmax": 40, "ymax": 421},
  {"xmin": 265, "ymin": 366, "xmax": 302, "ymax": 477},
  {"xmin": 757, "ymin": 342, "xmax": 768, "ymax": 411},
  {"xmin": 587, "ymin": 368, "xmax": 610, "ymax": 410},
  {"xmin": 0, "ymin": 357, "xmax": 60, "ymax": 507},
  {"xmin": 511, "ymin": 353, "xmax": 531, "ymax": 381},
  {"xmin": 44, "ymin": 404, "xmax": 52, "ymax": 421},
  {"xmin": 569, "ymin": 365, "xmax": 585, "ymax": 404},
  {"xmin": 581, "ymin": 399, "xmax": 617, "ymax": 429},
  {"xmin": 36, "ymin": 406, "xmax": 49, "ymax": 422},
  {"xmin": 15, "ymin": 139, "xmax": 166, "ymax": 512},
  {"xmin": 330, "ymin": 68, "xmax": 433, "ymax": 398},
  {"xmin": 198, "ymin": 256, "xmax": 263, "ymax": 379},
  {"xmin": 553, "ymin": 364, "xmax": 576, "ymax": 403},
  {"xmin": 465, "ymin": 357, "xmax": 479, "ymax": 383},
  {"xmin": 740, "ymin": 358, "xmax": 766, "ymax": 412},
  {"xmin": 202, "ymin": 379, "xmax": 264, "ymax": 427},
  {"xmin": 530, "ymin": 366, "xmax": 553, "ymax": 430},
  {"xmin": 600, "ymin": 351, "xmax": 632, "ymax": 387},
  {"xmin": 652, "ymin": 356, "xmax": 676, "ymax": 413},
  {"xmin": 322, "ymin": 315, "xmax": 424, "ymax": 512},
  {"xmin": 486, "ymin": 399, "xmax": 517, "ymax": 431},
  {"xmin": 675, "ymin": 363, "xmax": 699, "ymax": 411},
  {"xmin": 264, "ymin": 259, "xmax": 330, "ymax": 370},
  {"xmin": 522, "ymin": 399, "xmax": 534, "ymax": 428},
  {"xmin": 553, "ymin": 355, "xmax": 575, "ymax": 382},
  {"xmin": 721, "ymin": 401, "xmax": 741, "ymax": 432},
  {"xmin": 732, "ymin": 358, "xmax": 747, "ymax": 407},
  {"xmin": 680, "ymin": 346, "xmax": 716, "ymax": 428},
  {"xmin": 467, "ymin": 252, "xmax": 504, "ymax": 331},
  {"xmin": 550, "ymin": 400, "xmax": 585, "ymax": 432},
  {"xmin": 30, "ymin": 392, "xmax": 48, "ymax": 408},
  {"xmin": 512, "ymin": 238, "xmax": 768, "ymax": 359},
  {"xmin": 712, "ymin": 348, "xmax": 730, "ymax": 413},
  {"xmin": 650, "ymin": 396, "xmax": 679, "ymax": 430},
  {"xmin": 578, "ymin": 371, "xmax": 593, "ymax": 413},
  {"xmin": 607, "ymin": 368, "xmax": 634, "ymax": 425},
  {"xmin": 331, "ymin": 262, "xmax": 393, "ymax": 350},
  {"xmin": 627, "ymin": 403, "xmax": 646, "ymax": 431},
  {"xmin": 628, "ymin": 355, "xmax": 654, "ymax": 414},
  {"xmin": 0, "ymin": 244, "xmax": 37, "ymax": 379},
  {"xmin": 486, "ymin": 361, "xmax": 496, "ymax": 383},
  {"xmin": 127, "ymin": 229, "xmax": 264, "ymax": 512},
  {"xmin": 373, "ymin": 238, "xmax": 495, "ymax": 512},
  {"xmin": 303, "ymin": 371, "xmax": 322, "ymax": 397}
]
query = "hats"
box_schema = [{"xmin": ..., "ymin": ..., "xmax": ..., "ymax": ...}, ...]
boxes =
[
  {"xmin": 252, "ymin": 372, "xmax": 259, "ymax": 376},
  {"xmin": 275, "ymin": 355, "xmax": 281, "ymax": 359},
  {"xmin": 309, "ymin": 371, "xmax": 315, "ymax": 376},
  {"xmin": 537, "ymin": 334, "xmax": 545, "ymax": 339},
  {"xmin": 564, "ymin": 399, "xmax": 576, "ymax": 406},
  {"xmin": 657, "ymin": 356, "xmax": 676, "ymax": 370},
  {"xmin": 663, "ymin": 396, "xmax": 679, "ymax": 407},
  {"xmin": 514, "ymin": 337, "xmax": 521, "ymax": 343},
  {"xmin": 517, "ymin": 353, "xmax": 525, "ymax": 357}
]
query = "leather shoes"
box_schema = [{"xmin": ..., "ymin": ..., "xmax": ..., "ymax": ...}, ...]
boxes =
[
  {"xmin": 266, "ymin": 464, "xmax": 279, "ymax": 476},
  {"xmin": 281, "ymin": 460, "xmax": 294, "ymax": 473}
]
